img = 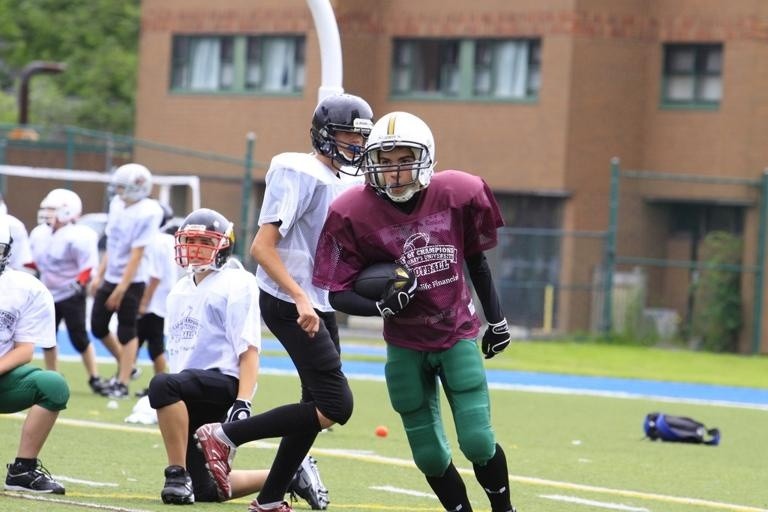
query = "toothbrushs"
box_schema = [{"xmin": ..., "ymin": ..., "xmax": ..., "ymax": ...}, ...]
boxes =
[{"xmin": 355, "ymin": 263, "xmax": 410, "ymax": 301}]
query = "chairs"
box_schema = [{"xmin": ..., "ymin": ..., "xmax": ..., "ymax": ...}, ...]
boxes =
[
  {"xmin": 5, "ymin": 463, "xmax": 65, "ymax": 494},
  {"xmin": 159, "ymin": 465, "xmax": 194, "ymax": 504},
  {"xmin": 194, "ymin": 423, "xmax": 233, "ymax": 499},
  {"xmin": 248, "ymin": 455, "xmax": 330, "ymax": 512},
  {"xmin": 88, "ymin": 367, "xmax": 153, "ymax": 396}
]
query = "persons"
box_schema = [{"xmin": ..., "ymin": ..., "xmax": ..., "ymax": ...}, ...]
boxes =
[
  {"xmin": 312, "ymin": 111, "xmax": 515, "ymax": 512},
  {"xmin": 1, "ymin": 217, "xmax": 70, "ymax": 494},
  {"xmin": 30, "ymin": 188, "xmax": 110, "ymax": 396},
  {"xmin": 0, "ymin": 197, "xmax": 36, "ymax": 274},
  {"xmin": 148, "ymin": 208, "xmax": 330, "ymax": 510},
  {"xmin": 135, "ymin": 200, "xmax": 186, "ymax": 396},
  {"xmin": 88, "ymin": 161, "xmax": 164, "ymax": 400},
  {"xmin": 194, "ymin": 92, "xmax": 373, "ymax": 512}
]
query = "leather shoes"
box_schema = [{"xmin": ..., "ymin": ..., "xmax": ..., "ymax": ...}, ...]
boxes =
[
  {"xmin": 376, "ymin": 272, "xmax": 417, "ymax": 320},
  {"xmin": 482, "ymin": 320, "xmax": 511, "ymax": 359},
  {"xmin": 223, "ymin": 399, "xmax": 251, "ymax": 424}
]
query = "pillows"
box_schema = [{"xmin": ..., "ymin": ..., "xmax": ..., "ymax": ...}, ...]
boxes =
[
  {"xmin": 364, "ymin": 111, "xmax": 435, "ymax": 203},
  {"xmin": 0, "ymin": 214, "xmax": 14, "ymax": 271},
  {"xmin": 175, "ymin": 208, "xmax": 235, "ymax": 271},
  {"xmin": 37, "ymin": 189, "xmax": 82, "ymax": 225},
  {"xmin": 310, "ymin": 94, "xmax": 374, "ymax": 176},
  {"xmin": 111, "ymin": 163, "xmax": 153, "ymax": 203}
]
